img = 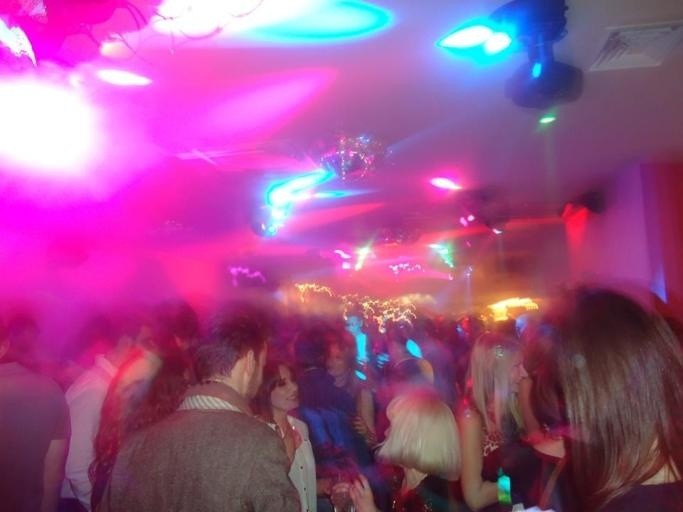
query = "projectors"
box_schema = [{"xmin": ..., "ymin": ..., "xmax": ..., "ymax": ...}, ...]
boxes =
[{"xmin": 504, "ymin": 59, "xmax": 585, "ymax": 110}]
